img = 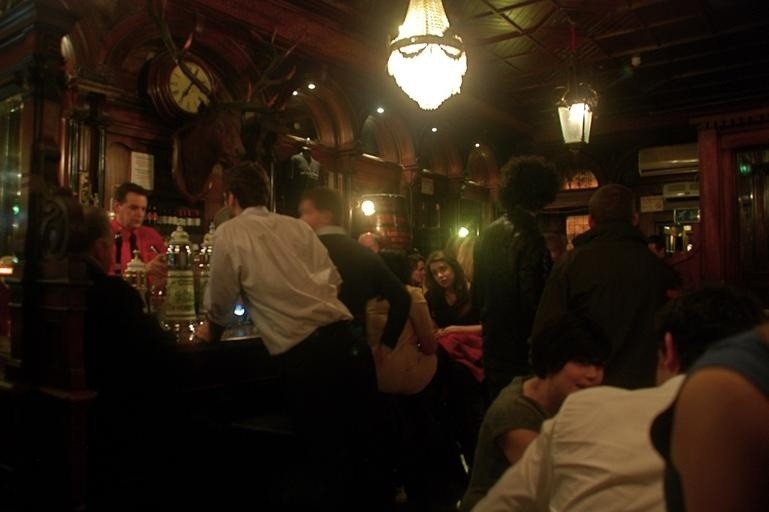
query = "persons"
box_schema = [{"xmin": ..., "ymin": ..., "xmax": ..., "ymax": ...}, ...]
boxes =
[
  {"xmin": 468, "ymin": 163, "xmax": 768, "ymax": 511},
  {"xmin": 81, "ymin": 162, "xmax": 484, "ymax": 511}
]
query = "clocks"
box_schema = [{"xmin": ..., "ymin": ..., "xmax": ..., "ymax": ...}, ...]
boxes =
[{"xmin": 148, "ymin": 48, "xmax": 220, "ymax": 127}]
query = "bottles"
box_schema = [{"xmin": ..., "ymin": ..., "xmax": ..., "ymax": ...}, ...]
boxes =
[
  {"xmin": 143, "ymin": 205, "xmax": 201, "ymax": 228},
  {"xmin": 115, "ymin": 242, "xmax": 213, "ymax": 321}
]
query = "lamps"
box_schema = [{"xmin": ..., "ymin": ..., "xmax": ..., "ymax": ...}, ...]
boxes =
[
  {"xmin": 556, "ymin": 29, "xmax": 599, "ymax": 145},
  {"xmin": 386, "ymin": 0, "xmax": 468, "ymax": 110}
]
